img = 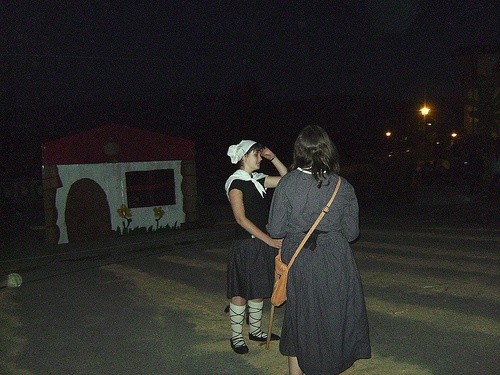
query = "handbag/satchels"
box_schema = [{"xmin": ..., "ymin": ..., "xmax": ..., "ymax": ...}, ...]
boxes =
[{"xmin": 270, "ymin": 253, "xmax": 289, "ymax": 306}]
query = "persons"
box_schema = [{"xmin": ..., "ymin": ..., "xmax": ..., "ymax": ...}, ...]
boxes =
[
  {"xmin": 225, "ymin": 140, "xmax": 288, "ymax": 355},
  {"xmin": 264, "ymin": 124, "xmax": 373, "ymax": 375}
]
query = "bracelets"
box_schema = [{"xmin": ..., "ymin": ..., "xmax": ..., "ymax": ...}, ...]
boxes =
[{"xmin": 271, "ymin": 153, "xmax": 276, "ymax": 162}]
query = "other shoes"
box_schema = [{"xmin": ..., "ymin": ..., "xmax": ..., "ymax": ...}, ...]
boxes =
[
  {"xmin": 230, "ymin": 337, "xmax": 248, "ymax": 354},
  {"xmin": 249, "ymin": 330, "xmax": 280, "ymax": 341}
]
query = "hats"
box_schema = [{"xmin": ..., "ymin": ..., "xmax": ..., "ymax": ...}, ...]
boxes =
[{"xmin": 227, "ymin": 140, "xmax": 257, "ymax": 164}]
